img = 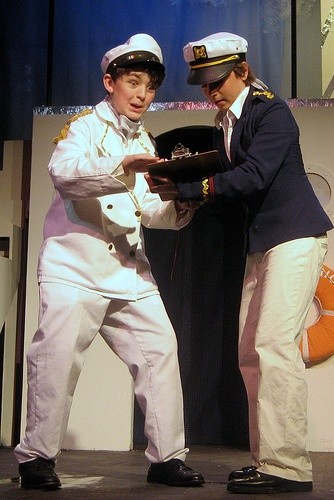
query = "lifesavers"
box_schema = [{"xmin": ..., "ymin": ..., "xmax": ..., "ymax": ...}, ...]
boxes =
[{"xmin": 300, "ymin": 263, "xmax": 334, "ymax": 366}]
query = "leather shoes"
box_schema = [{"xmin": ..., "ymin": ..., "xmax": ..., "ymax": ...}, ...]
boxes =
[
  {"xmin": 146, "ymin": 459, "xmax": 205, "ymax": 487},
  {"xmin": 226, "ymin": 471, "xmax": 313, "ymax": 495},
  {"xmin": 18, "ymin": 456, "xmax": 62, "ymax": 490},
  {"xmin": 227, "ymin": 466, "xmax": 258, "ymax": 482}
]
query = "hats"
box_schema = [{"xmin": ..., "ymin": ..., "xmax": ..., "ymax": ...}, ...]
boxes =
[
  {"xmin": 183, "ymin": 32, "xmax": 249, "ymax": 85},
  {"xmin": 100, "ymin": 33, "xmax": 167, "ymax": 76}
]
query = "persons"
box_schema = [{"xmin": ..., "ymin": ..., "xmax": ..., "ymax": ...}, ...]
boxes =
[
  {"xmin": 144, "ymin": 32, "xmax": 334, "ymax": 494},
  {"xmin": 12, "ymin": 32, "xmax": 206, "ymax": 490}
]
